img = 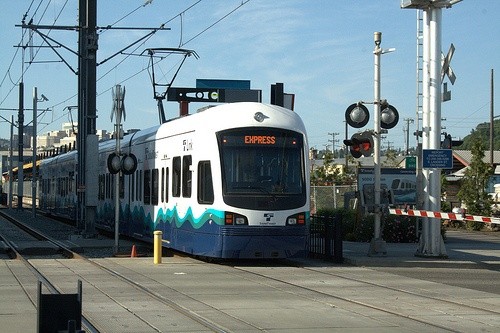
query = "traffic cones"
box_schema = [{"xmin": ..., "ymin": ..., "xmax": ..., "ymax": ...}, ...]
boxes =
[{"xmin": 130, "ymin": 244, "xmax": 137, "ymax": 258}]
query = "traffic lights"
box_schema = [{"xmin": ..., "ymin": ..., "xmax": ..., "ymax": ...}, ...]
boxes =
[{"xmin": 344, "ymin": 131, "xmax": 373, "ymax": 159}]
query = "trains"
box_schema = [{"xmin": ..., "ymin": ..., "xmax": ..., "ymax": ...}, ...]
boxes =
[{"xmin": 4, "ymin": 101, "xmax": 313, "ymax": 262}]
